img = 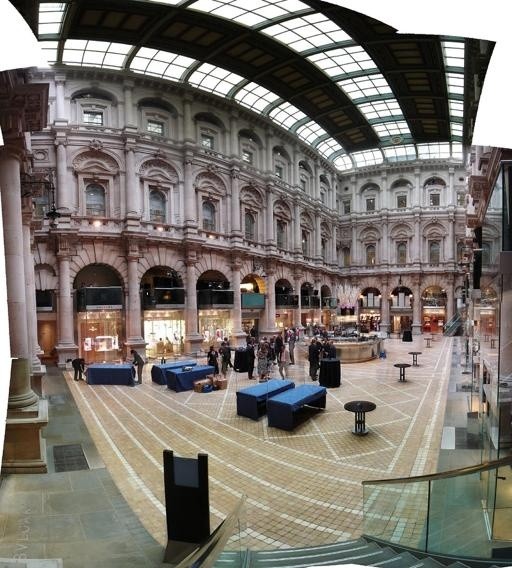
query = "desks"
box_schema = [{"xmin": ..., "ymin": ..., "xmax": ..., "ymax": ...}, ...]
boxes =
[
  {"xmin": 236, "ymin": 380, "xmax": 294, "ymax": 419},
  {"xmin": 166, "ymin": 365, "xmax": 215, "ymax": 391},
  {"xmin": 394, "ymin": 364, "xmax": 411, "ymax": 382},
  {"xmin": 424, "ymin": 333, "xmax": 439, "ymax": 348},
  {"xmin": 150, "ymin": 361, "xmax": 196, "ymax": 385},
  {"xmin": 344, "ymin": 401, "xmax": 376, "ymax": 436},
  {"xmin": 266, "ymin": 384, "xmax": 326, "ymax": 431},
  {"xmin": 408, "ymin": 352, "xmax": 422, "ymax": 367}
]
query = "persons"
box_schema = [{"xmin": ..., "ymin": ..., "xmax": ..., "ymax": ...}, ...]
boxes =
[
  {"xmin": 219, "ymin": 338, "xmax": 238, "ymax": 376},
  {"xmin": 245, "ymin": 326, "xmax": 299, "ymax": 382},
  {"xmin": 206, "ymin": 345, "xmax": 218, "ymax": 375},
  {"xmin": 131, "ymin": 350, "xmax": 145, "ymax": 384},
  {"xmin": 73, "ymin": 358, "xmax": 84, "ymax": 381},
  {"xmin": 309, "ymin": 337, "xmax": 336, "ymax": 381},
  {"xmin": 314, "ymin": 326, "xmax": 328, "ymax": 337}
]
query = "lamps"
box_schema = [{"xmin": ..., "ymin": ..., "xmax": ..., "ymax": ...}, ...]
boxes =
[
  {"xmin": 86, "ymin": 364, "xmax": 136, "ymax": 386},
  {"xmin": 21, "ymin": 178, "xmax": 61, "ymax": 221}
]
